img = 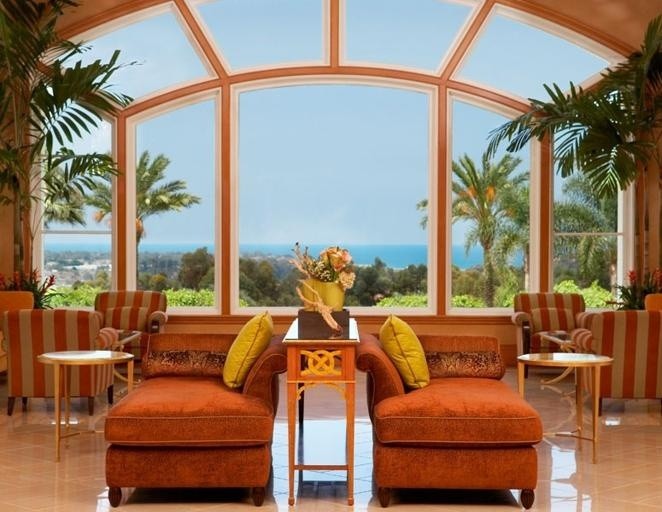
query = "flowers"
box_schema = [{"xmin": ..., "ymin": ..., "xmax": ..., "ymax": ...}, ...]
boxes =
[
  {"xmin": 291, "ymin": 240, "xmax": 355, "ymax": 291},
  {"xmin": 0, "ymin": 271, "xmax": 66, "ymax": 310},
  {"xmin": 605, "ymin": 269, "xmax": 662, "ymax": 311}
]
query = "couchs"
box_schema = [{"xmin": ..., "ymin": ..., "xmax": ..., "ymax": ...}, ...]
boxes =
[
  {"xmin": 0, "ymin": 290, "xmax": 37, "ymax": 374},
  {"xmin": 92, "ymin": 289, "xmax": 166, "ymax": 364},
  {"xmin": 0, "ymin": 309, "xmax": 121, "ymax": 416},
  {"xmin": 570, "ymin": 308, "xmax": 662, "ymax": 417},
  {"xmin": 106, "ymin": 328, "xmax": 287, "ymax": 509},
  {"xmin": 354, "ymin": 331, "xmax": 543, "ymax": 511},
  {"xmin": 511, "ymin": 291, "xmax": 587, "ymax": 379}
]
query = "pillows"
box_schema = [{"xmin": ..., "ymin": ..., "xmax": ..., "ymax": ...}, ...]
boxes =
[
  {"xmin": 221, "ymin": 309, "xmax": 274, "ymax": 389},
  {"xmin": 141, "ymin": 348, "xmax": 227, "ymax": 377},
  {"xmin": 378, "ymin": 313, "xmax": 431, "ymax": 390},
  {"xmin": 421, "ymin": 347, "xmax": 506, "ymax": 380}
]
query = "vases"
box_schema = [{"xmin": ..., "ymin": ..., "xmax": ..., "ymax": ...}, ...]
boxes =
[{"xmin": 301, "ymin": 280, "xmax": 344, "ymax": 313}]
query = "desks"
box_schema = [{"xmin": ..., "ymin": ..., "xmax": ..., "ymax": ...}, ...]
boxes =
[
  {"xmin": 282, "ymin": 317, "xmax": 361, "ymax": 508},
  {"xmin": 36, "ymin": 350, "xmax": 134, "ymax": 463},
  {"xmin": 514, "ymin": 352, "xmax": 614, "ymax": 464}
]
